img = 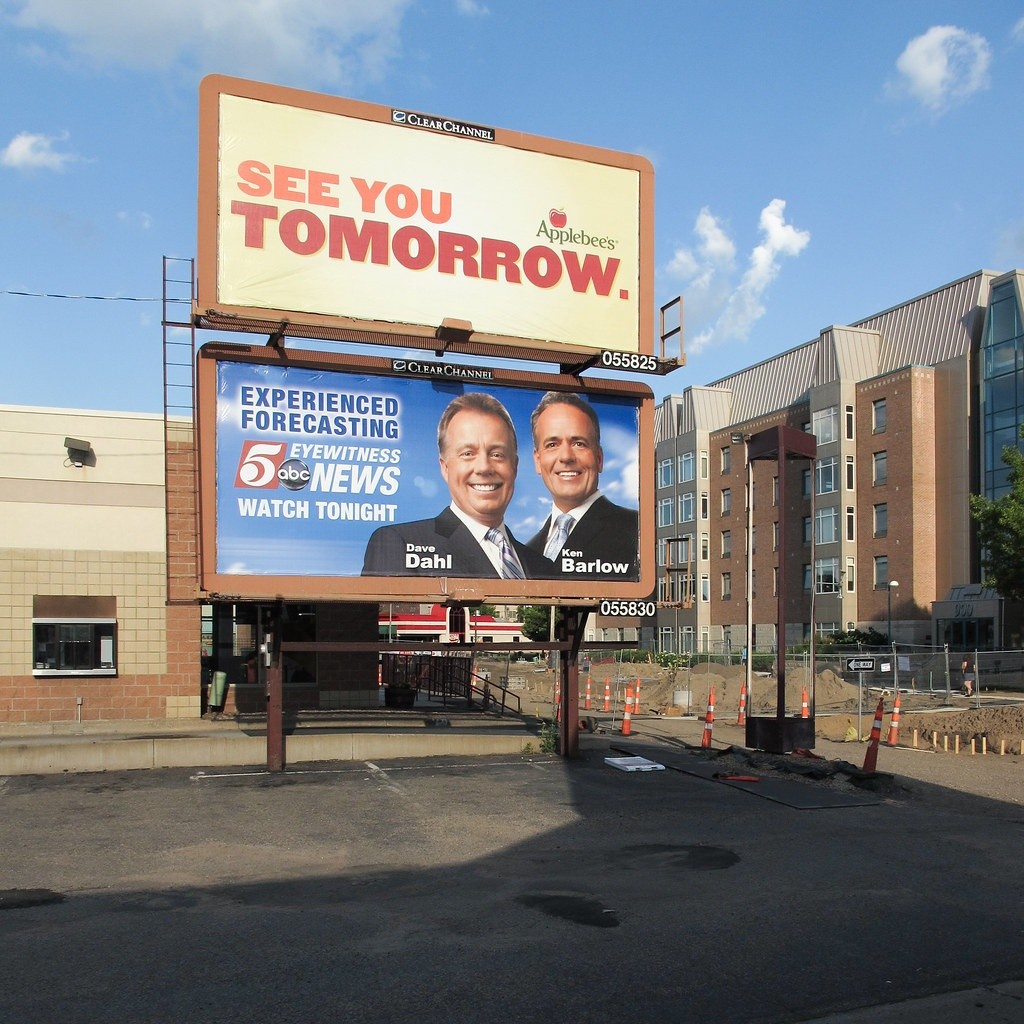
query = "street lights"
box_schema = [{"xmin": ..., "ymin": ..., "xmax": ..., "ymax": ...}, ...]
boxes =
[{"xmin": 887, "ymin": 580, "xmax": 900, "ymax": 653}]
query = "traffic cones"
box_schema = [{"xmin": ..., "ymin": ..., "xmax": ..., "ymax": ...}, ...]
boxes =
[
  {"xmin": 685, "ymin": 687, "xmax": 720, "ymax": 751},
  {"xmin": 798, "ymin": 687, "xmax": 810, "ymax": 718},
  {"xmin": 471, "ymin": 666, "xmax": 479, "ymax": 687},
  {"xmin": 597, "ymin": 676, "xmax": 615, "ymax": 712},
  {"xmin": 881, "ymin": 691, "xmax": 906, "ymax": 749},
  {"xmin": 858, "ymin": 696, "xmax": 887, "ymax": 777},
  {"xmin": 554, "ymin": 677, "xmax": 561, "ymax": 723},
  {"xmin": 612, "ymin": 681, "xmax": 640, "ymax": 736},
  {"xmin": 726, "ymin": 682, "xmax": 747, "ymax": 726},
  {"xmin": 629, "ymin": 677, "xmax": 646, "ymax": 716},
  {"xmin": 583, "ymin": 674, "xmax": 595, "ymax": 710}
]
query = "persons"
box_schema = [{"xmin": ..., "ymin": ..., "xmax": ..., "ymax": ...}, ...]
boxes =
[
  {"xmin": 524, "ymin": 390, "xmax": 638, "ymax": 580},
  {"xmin": 742, "ymin": 646, "xmax": 747, "ymax": 666},
  {"xmin": 360, "ymin": 393, "xmax": 559, "ymax": 580},
  {"xmin": 960, "ymin": 655, "xmax": 975, "ymax": 697},
  {"xmin": 583, "ymin": 657, "xmax": 592, "ymax": 672}
]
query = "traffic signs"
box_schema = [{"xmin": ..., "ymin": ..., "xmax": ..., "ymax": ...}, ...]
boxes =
[{"xmin": 845, "ymin": 657, "xmax": 876, "ymax": 673}]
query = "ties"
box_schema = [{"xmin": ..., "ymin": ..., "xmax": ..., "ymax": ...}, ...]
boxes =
[
  {"xmin": 543, "ymin": 514, "xmax": 575, "ymax": 561},
  {"xmin": 485, "ymin": 528, "xmax": 525, "ymax": 580}
]
